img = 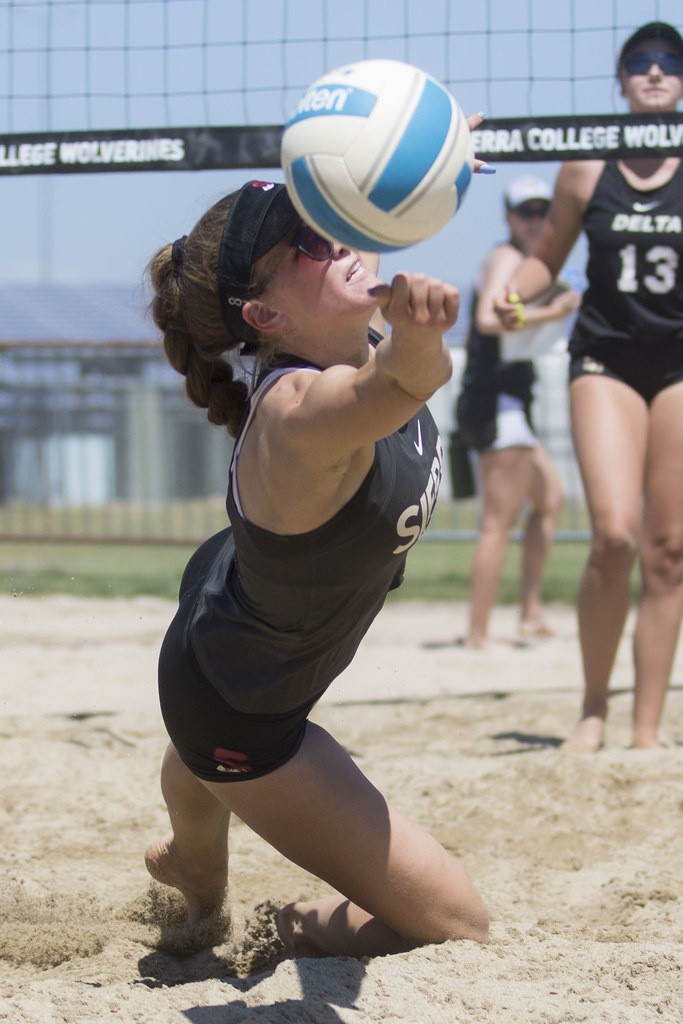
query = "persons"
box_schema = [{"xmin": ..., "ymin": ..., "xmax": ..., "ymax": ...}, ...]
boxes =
[
  {"xmin": 449, "ymin": 183, "xmax": 583, "ymax": 651},
  {"xmin": 500, "ymin": 14, "xmax": 683, "ymax": 759},
  {"xmin": 135, "ymin": 178, "xmax": 498, "ymax": 962}
]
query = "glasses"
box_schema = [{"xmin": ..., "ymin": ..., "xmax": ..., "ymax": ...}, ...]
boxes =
[
  {"xmin": 510, "ymin": 204, "xmax": 551, "ymax": 219},
  {"xmin": 245, "ymin": 218, "xmax": 333, "ymax": 302},
  {"xmin": 622, "ymin": 50, "xmax": 683, "ymax": 77}
]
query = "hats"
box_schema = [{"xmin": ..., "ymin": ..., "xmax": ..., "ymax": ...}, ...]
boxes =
[
  {"xmin": 219, "ymin": 181, "xmax": 302, "ymax": 354},
  {"xmin": 504, "ymin": 174, "xmax": 554, "ymax": 209},
  {"xmin": 620, "ymin": 22, "xmax": 682, "ymax": 59}
]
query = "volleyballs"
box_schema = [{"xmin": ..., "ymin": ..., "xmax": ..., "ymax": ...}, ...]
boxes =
[{"xmin": 279, "ymin": 57, "xmax": 474, "ymax": 254}]
